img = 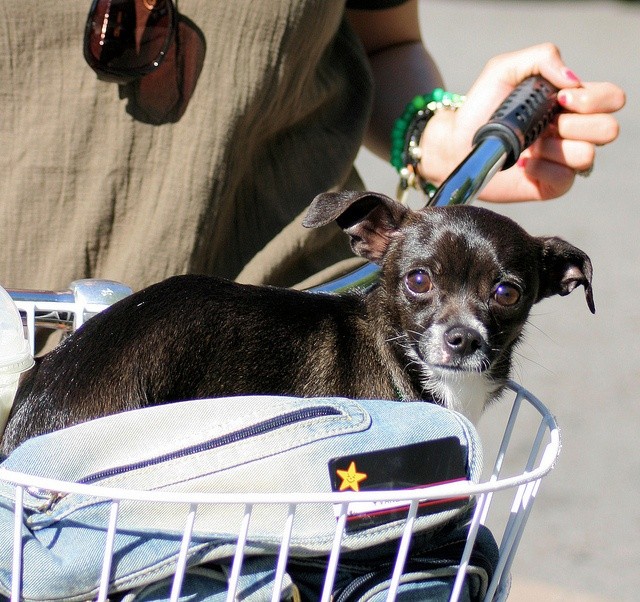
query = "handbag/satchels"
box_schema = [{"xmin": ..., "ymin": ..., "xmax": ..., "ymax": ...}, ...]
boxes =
[{"xmin": 3, "ymin": 390, "xmax": 506, "ymax": 602}]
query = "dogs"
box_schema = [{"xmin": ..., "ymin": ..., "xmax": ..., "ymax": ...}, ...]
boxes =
[{"xmin": 0, "ymin": 192, "xmax": 595, "ymax": 465}]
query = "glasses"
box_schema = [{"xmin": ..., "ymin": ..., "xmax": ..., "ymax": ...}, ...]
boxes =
[{"xmin": 82, "ymin": 1, "xmax": 175, "ymax": 85}]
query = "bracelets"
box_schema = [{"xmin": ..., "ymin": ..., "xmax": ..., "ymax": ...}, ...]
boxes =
[
  {"xmin": 401, "ymin": 100, "xmax": 459, "ymax": 188},
  {"xmin": 389, "ymin": 88, "xmax": 465, "ymax": 194}
]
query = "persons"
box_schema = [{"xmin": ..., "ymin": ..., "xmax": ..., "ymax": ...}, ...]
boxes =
[{"xmin": 0, "ymin": 1, "xmax": 626, "ymax": 359}]
